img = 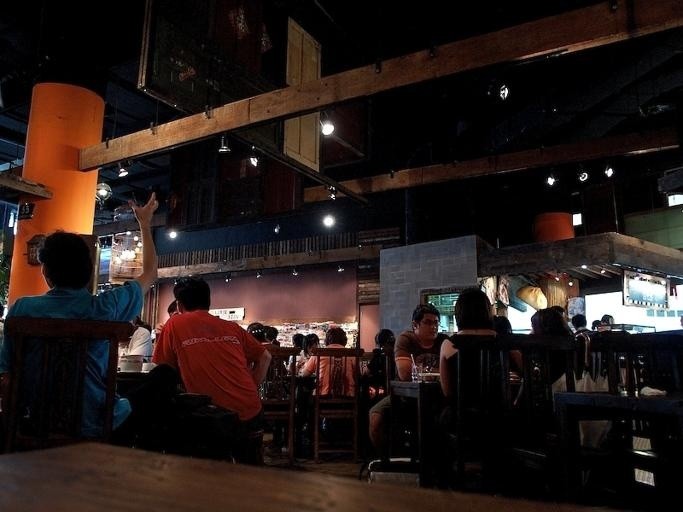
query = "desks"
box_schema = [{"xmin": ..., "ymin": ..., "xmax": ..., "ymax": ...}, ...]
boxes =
[
  {"xmin": 389, "ymin": 380, "xmax": 441, "ymax": 459},
  {"xmin": 0, "ymin": 443, "xmax": 613, "ymax": 512}
]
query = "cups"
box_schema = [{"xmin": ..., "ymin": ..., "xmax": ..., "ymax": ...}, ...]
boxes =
[
  {"xmin": 142, "ymin": 362, "xmax": 151, "ymax": 371},
  {"xmin": 410, "ymin": 359, "xmax": 424, "ymax": 384},
  {"xmin": 632, "ymin": 356, "xmax": 644, "ymax": 397},
  {"xmin": 618, "ymin": 356, "xmax": 627, "ymax": 394}
]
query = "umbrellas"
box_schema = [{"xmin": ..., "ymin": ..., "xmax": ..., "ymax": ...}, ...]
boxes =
[{"xmin": 552, "ymin": 333, "xmax": 609, "ymax": 485}]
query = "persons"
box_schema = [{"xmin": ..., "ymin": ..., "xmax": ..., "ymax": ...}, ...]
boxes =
[
  {"xmin": 369, "ymin": 304, "xmax": 451, "ymax": 459},
  {"xmin": 440, "ymin": 288, "xmax": 497, "ymax": 398},
  {"xmin": 369, "ymin": 329, "xmax": 395, "ymax": 399},
  {"xmin": 513, "ymin": 309, "xmax": 573, "ymax": 407},
  {"xmin": 572, "ymin": 314, "xmax": 591, "ymax": 335},
  {"xmin": 485, "ymin": 301, "xmax": 522, "ymax": 388},
  {"xmin": 265, "ymin": 327, "xmax": 356, "ymax": 457},
  {"xmin": 117, "ymin": 322, "xmax": 152, "ymax": 363},
  {"xmin": 247, "ymin": 323, "xmax": 290, "ymax": 401},
  {"xmin": 363, "ymin": 348, "xmax": 386, "ymax": 387},
  {"xmin": 153, "ymin": 277, "xmax": 271, "ymax": 465},
  {"xmin": 300, "ymin": 334, "xmax": 321, "ymax": 375},
  {"xmin": 288, "ymin": 334, "xmax": 303, "ymax": 373},
  {"xmin": 266, "ymin": 327, "xmax": 278, "ymax": 342},
  {"xmin": 154, "ymin": 298, "xmax": 179, "ymax": 349},
  {"xmin": 0, "ymin": 193, "xmax": 159, "ymax": 444}
]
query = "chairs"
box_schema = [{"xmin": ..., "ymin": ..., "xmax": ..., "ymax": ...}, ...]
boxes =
[
  {"xmin": 246, "ymin": 346, "xmax": 301, "ymax": 467},
  {"xmin": 0, "ymin": 316, "xmax": 134, "ymax": 454},
  {"xmin": 311, "ymin": 348, "xmax": 364, "ymax": 464},
  {"xmin": 439, "ymin": 332, "xmax": 683, "ymax": 508}
]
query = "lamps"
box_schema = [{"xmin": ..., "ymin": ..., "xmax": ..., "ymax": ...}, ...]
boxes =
[
  {"xmin": 320, "ymin": 123, "xmax": 335, "ymax": 137},
  {"xmin": 337, "ymin": 264, "xmax": 345, "ymax": 273},
  {"xmin": 292, "ymin": 269, "xmax": 297, "ymax": 276},
  {"xmin": 256, "ymin": 272, "xmax": 262, "ymax": 278},
  {"xmin": 92, "ymin": 180, "xmax": 112, "ymax": 207},
  {"xmin": 223, "ymin": 273, "xmax": 231, "ymax": 283},
  {"xmin": 250, "ymin": 157, "xmax": 259, "ymax": 169},
  {"xmin": 667, "ymin": 194, "xmax": 683, "ymax": 209},
  {"xmin": 218, "ymin": 145, "xmax": 231, "ymax": 155},
  {"xmin": 572, "ymin": 213, "xmax": 583, "ymax": 228},
  {"xmin": 577, "ymin": 172, "xmax": 589, "ymax": 183},
  {"xmin": 546, "ymin": 176, "xmax": 556, "ymax": 189},
  {"xmin": 108, "ymin": 203, "xmax": 148, "ymax": 282},
  {"xmin": 603, "ymin": 166, "xmax": 615, "ymax": 181},
  {"xmin": 499, "ymin": 85, "xmax": 510, "ymax": 100},
  {"xmin": 321, "ymin": 215, "xmax": 335, "ymax": 226}
]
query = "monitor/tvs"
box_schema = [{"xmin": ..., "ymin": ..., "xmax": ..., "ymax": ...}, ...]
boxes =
[{"xmin": 622, "ymin": 271, "xmax": 671, "ymax": 311}]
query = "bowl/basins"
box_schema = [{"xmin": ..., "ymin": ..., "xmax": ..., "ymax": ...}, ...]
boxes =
[
  {"xmin": 419, "ymin": 372, "xmax": 441, "ymax": 382},
  {"xmin": 121, "ymin": 354, "xmax": 145, "ymax": 360},
  {"xmin": 120, "ymin": 359, "xmax": 143, "ymax": 372}
]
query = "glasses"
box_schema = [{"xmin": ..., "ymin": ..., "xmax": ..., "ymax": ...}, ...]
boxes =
[{"xmin": 420, "ymin": 319, "xmax": 440, "ymax": 326}]
